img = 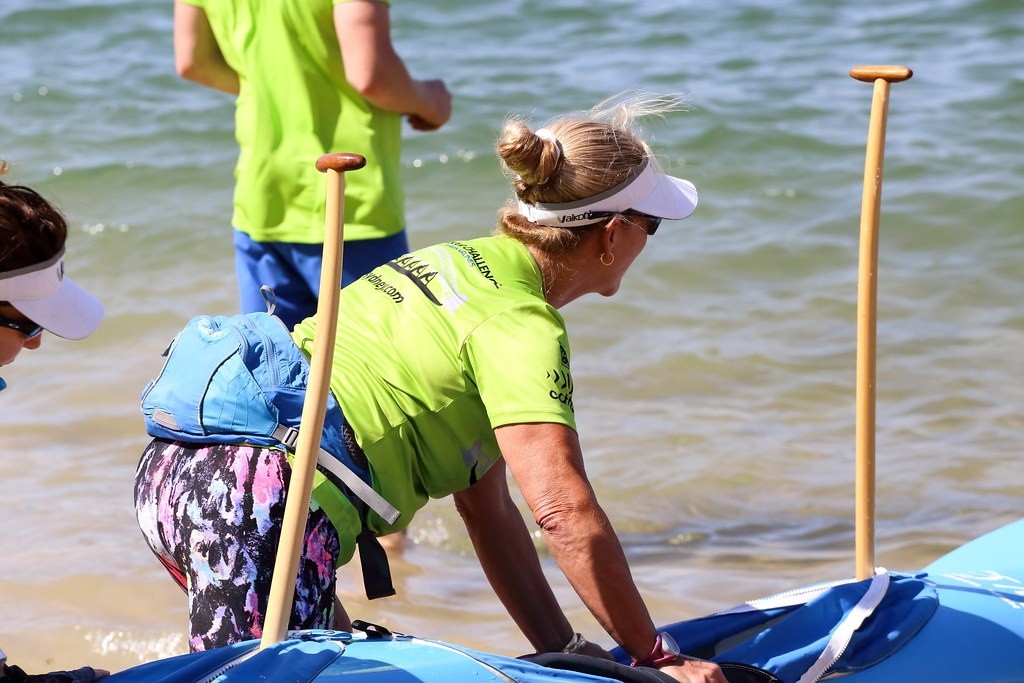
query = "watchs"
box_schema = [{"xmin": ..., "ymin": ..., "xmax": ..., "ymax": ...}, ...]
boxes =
[{"xmin": 627, "ymin": 631, "xmax": 680, "ymax": 672}]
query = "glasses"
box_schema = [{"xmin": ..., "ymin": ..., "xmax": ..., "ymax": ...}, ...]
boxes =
[
  {"xmin": 586, "ymin": 211, "xmax": 663, "ymax": 236},
  {"xmin": 0, "ymin": 316, "xmax": 45, "ymax": 337}
]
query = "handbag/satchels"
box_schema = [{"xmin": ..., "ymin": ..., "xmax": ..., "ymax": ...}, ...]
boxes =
[{"xmin": 139, "ymin": 308, "xmax": 347, "ymax": 467}]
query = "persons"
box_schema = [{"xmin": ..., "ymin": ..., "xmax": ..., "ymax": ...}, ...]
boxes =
[
  {"xmin": 1, "ymin": 181, "xmax": 110, "ymax": 683},
  {"xmin": 172, "ymin": 1, "xmax": 452, "ymax": 550},
  {"xmin": 133, "ymin": 118, "xmax": 730, "ymax": 683}
]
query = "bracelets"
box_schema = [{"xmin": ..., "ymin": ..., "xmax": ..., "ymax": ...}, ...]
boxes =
[{"xmin": 561, "ymin": 633, "xmax": 587, "ymax": 653}]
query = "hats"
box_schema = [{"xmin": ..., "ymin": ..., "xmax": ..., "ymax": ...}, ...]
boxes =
[
  {"xmin": 514, "ymin": 137, "xmax": 699, "ymax": 229},
  {"xmin": 0, "ymin": 237, "xmax": 106, "ymax": 339}
]
